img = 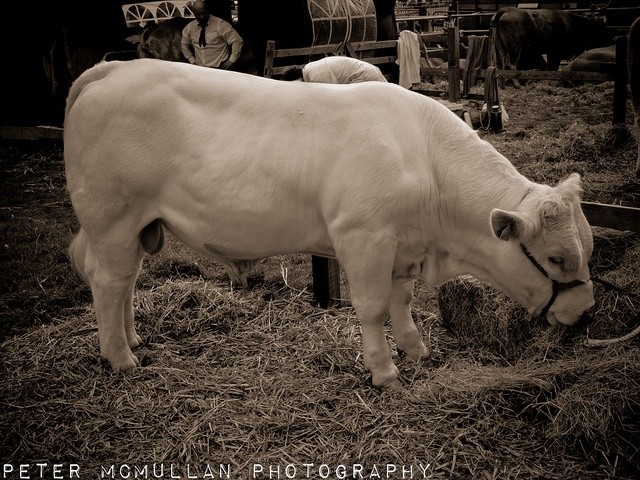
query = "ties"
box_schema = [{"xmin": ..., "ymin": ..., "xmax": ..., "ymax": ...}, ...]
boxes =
[{"xmin": 199, "ymin": 25, "xmax": 206, "ymax": 47}]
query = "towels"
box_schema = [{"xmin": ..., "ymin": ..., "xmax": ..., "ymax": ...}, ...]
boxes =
[
  {"xmin": 393, "ymin": 29, "xmax": 421, "ymax": 90},
  {"xmin": 486, "ymin": 65, "xmax": 495, "ymax": 115},
  {"xmin": 461, "ymin": 35, "xmax": 488, "ymax": 97}
]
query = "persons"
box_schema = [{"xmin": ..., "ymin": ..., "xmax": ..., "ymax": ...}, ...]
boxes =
[
  {"xmin": 281, "ymin": 55, "xmax": 390, "ymax": 85},
  {"xmin": 179, "ymin": 0, "xmax": 244, "ymax": 70}
]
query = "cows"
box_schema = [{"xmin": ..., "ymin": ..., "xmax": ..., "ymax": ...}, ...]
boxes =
[
  {"xmin": 64, "ymin": 58, "xmax": 596, "ymax": 391},
  {"xmin": 628, "ymin": 17, "xmax": 640, "ymax": 178},
  {"xmin": 491, "ymin": 1, "xmax": 611, "ymax": 90}
]
